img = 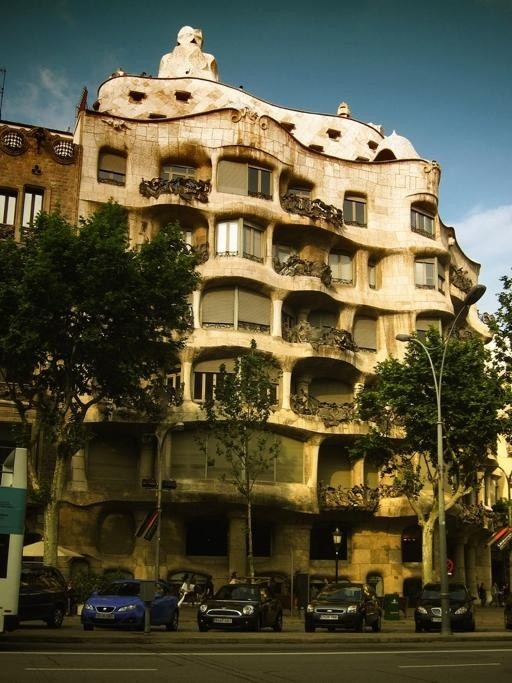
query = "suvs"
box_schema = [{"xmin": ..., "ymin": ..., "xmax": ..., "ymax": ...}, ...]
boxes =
[{"xmin": 17, "ymin": 560, "xmax": 72, "ymax": 628}]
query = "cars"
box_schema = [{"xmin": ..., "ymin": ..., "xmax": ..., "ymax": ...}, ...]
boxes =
[
  {"xmin": 80, "ymin": 578, "xmax": 181, "ymax": 633},
  {"xmin": 197, "ymin": 582, "xmax": 283, "ymax": 632},
  {"xmin": 503, "ymin": 591, "xmax": 511, "ymax": 631},
  {"xmin": 303, "ymin": 581, "xmax": 383, "ymax": 634},
  {"xmin": 412, "ymin": 581, "xmax": 477, "ymax": 634}
]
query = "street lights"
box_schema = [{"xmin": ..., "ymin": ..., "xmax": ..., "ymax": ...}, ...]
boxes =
[
  {"xmin": 395, "ymin": 282, "xmax": 487, "ymax": 636},
  {"xmin": 153, "ymin": 420, "xmax": 185, "ymax": 581},
  {"xmin": 332, "ymin": 527, "xmax": 343, "ymax": 582}
]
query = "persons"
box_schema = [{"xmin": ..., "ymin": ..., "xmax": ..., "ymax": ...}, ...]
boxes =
[
  {"xmin": 176, "ymin": 571, "xmax": 241, "ymax": 607},
  {"xmin": 478, "ymin": 582, "xmax": 509, "ymax": 609}
]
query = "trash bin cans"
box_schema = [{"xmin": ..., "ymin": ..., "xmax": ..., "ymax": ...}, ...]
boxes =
[{"xmin": 384, "ymin": 594, "xmax": 400, "ymax": 620}]
